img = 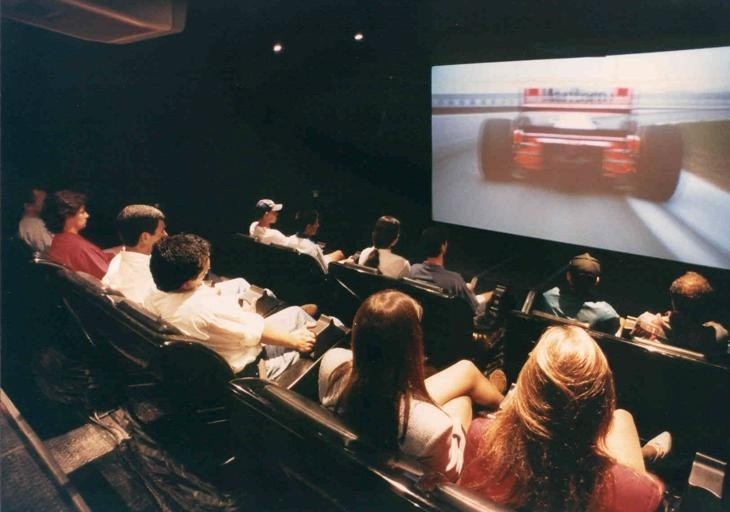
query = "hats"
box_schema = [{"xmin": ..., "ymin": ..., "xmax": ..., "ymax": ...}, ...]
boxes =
[
  {"xmin": 253, "ymin": 196, "xmax": 285, "ymax": 214},
  {"xmin": 566, "ymin": 252, "xmax": 602, "ymax": 288}
]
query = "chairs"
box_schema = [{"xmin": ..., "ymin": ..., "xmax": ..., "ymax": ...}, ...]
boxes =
[{"xmin": 0, "ymin": 230, "xmax": 730, "ymax": 512}]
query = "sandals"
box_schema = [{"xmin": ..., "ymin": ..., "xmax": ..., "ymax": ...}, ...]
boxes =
[{"xmin": 643, "ymin": 429, "xmax": 674, "ymax": 467}]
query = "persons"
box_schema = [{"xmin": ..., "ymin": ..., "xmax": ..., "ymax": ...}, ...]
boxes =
[
  {"xmin": 358, "ymin": 215, "xmax": 411, "ymax": 279},
  {"xmin": 410, "ymin": 227, "xmax": 494, "ymax": 317},
  {"xmin": 279, "ymin": 207, "xmax": 357, "ymax": 275},
  {"xmin": 459, "ymin": 323, "xmax": 674, "ymax": 511},
  {"xmin": 16, "ymin": 186, "xmax": 109, "ymax": 259},
  {"xmin": 138, "ymin": 233, "xmax": 344, "ymax": 381},
  {"xmin": 317, "ymin": 287, "xmax": 509, "ymax": 483},
  {"xmin": 42, "ymin": 188, "xmax": 125, "ymax": 280},
  {"xmin": 541, "ymin": 251, "xmax": 624, "ymax": 337},
  {"xmin": 624, "ymin": 272, "xmax": 729, "ymax": 360},
  {"xmin": 101, "ymin": 203, "xmax": 253, "ymax": 303},
  {"xmin": 249, "ymin": 199, "xmax": 288, "ymax": 245}
]
